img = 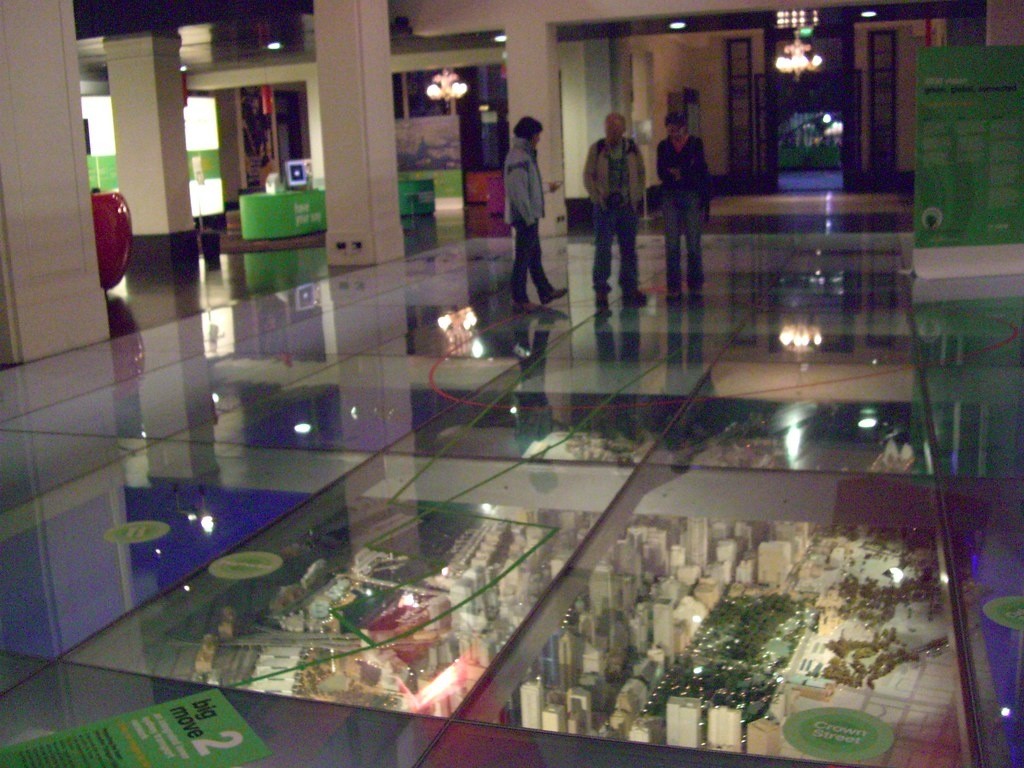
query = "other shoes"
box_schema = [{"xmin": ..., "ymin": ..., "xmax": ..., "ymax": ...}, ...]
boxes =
[
  {"xmin": 621, "ymin": 291, "xmax": 646, "ymax": 308},
  {"xmin": 596, "ymin": 290, "xmax": 609, "ymax": 308},
  {"xmin": 665, "ymin": 291, "xmax": 682, "ymax": 307},
  {"xmin": 539, "ymin": 288, "xmax": 569, "ymax": 305},
  {"xmin": 688, "ymin": 295, "xmax": 704, "ymax": 309},
  {"xmin": 511, "ymin": 300, "xmax": 541, "ymax": 313}
]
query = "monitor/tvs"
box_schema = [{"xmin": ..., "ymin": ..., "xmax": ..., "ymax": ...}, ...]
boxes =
[{"xmin": 287, "ymin": 159, "xmax": 312, "ymax": 186}]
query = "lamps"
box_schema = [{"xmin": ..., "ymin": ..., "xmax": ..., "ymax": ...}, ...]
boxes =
[
  {"xmin": 426, "ymin": 69, "xmax": 468, "ymax": 103},
  {"xmin": 773, "ymin": 28, "xmax": 823, "ymax": 79}
]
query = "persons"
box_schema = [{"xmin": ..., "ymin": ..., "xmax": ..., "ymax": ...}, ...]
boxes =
[
  {"xmin": 584, "ymin": 113, "xmax": 647, "ymax": 302},
  {"xmin": 659, "ymin": 295, "xmax": 711, "ymax": 474},
  {"xmin": 589, "ymin": 305, "xmax": 641, "ymax": 466},
  {"xmin": 656, "ymin": 112, "xmax": 709, "ymax": 299},
  {"xmin": 511, "ymin": 308, "xmax": 568, "ymax": 492},
  {"xmin": 504, "ymin": 116, "xmax": 568, "ymax": 311}
]
